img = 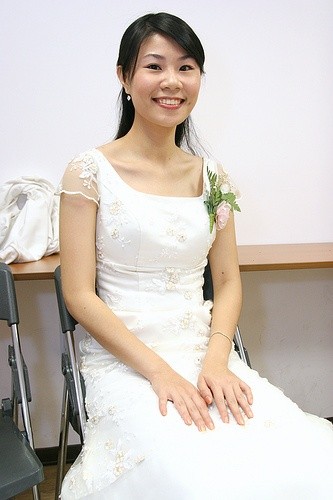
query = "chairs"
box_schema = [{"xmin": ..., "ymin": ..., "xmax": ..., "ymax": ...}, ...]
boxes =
[
  {"xmin": 56, "ymin": 265, "xmax": 253, "ymax": 500},
  {"xmin": 0, "ymin": 262, "xmax": 45, "ymax": 500}
]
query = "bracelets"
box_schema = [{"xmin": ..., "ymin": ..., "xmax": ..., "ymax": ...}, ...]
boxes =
[{"xmin": 209, "ymin": 331, "xmax": 233, "ymax": 352}]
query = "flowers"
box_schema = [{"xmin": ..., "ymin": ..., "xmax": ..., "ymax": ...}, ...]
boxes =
[{"xmin": 203, "ymin": 166, "xmax": 241, "ymax": 234}]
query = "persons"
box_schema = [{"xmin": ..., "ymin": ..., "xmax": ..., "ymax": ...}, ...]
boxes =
[{"xmin": 54, "ymin": 12, "xmax": 333, "ymax": 500}]
